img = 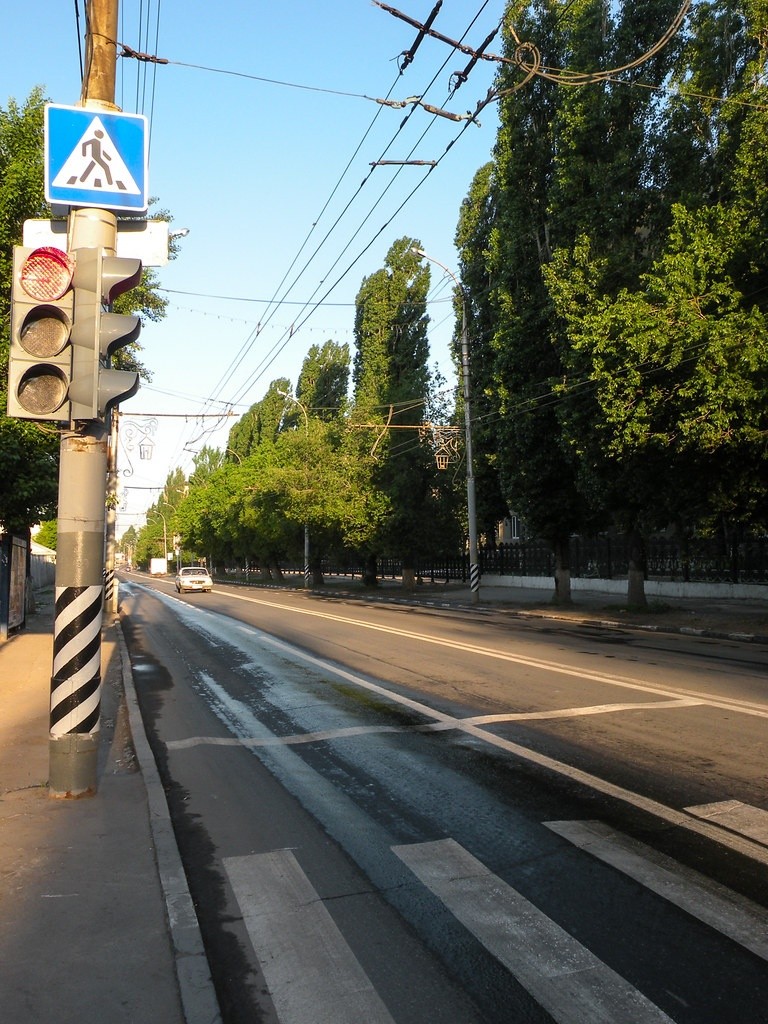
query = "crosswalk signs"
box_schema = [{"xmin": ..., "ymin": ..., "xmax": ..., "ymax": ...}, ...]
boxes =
[{"xmin": 44, "ymin": 102, "xmax": 153, "ymax": 217}]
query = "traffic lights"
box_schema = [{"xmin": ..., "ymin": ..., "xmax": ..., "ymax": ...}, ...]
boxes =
[
  {"xmin": 9, "ymin": 244, "xmax": 75, "ymax": 422},
  {"xmin": 75, "ymin": 245, "xmax": 144, "ymax": 429}
]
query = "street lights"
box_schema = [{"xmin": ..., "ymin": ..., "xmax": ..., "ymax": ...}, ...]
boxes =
[
  {"xmin": 217, "ymin": 445, "xmax": 251, "ymax": 582},
  {"xmin": 278, "ymin": 390, "xmax": 311, "ymax": 589},
  {"xmin": 153, "ymin": 510, "xmax": 168, "ymax": 561},
  {"xmin": 410, "ymin": 246, "xmax": 485, "ymax": 604},
  {"xmin": 191, "ymin": 474, "xmax": 214, "ymax": 579},
  {"xmin": 163, "ymin": 502, "xmax": 181, "ymax": 573}
]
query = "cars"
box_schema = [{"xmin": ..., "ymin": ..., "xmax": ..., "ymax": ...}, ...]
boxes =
[{"xmin": 176, "ymin": 567, "xmax": 214, "ymax": 595}]
